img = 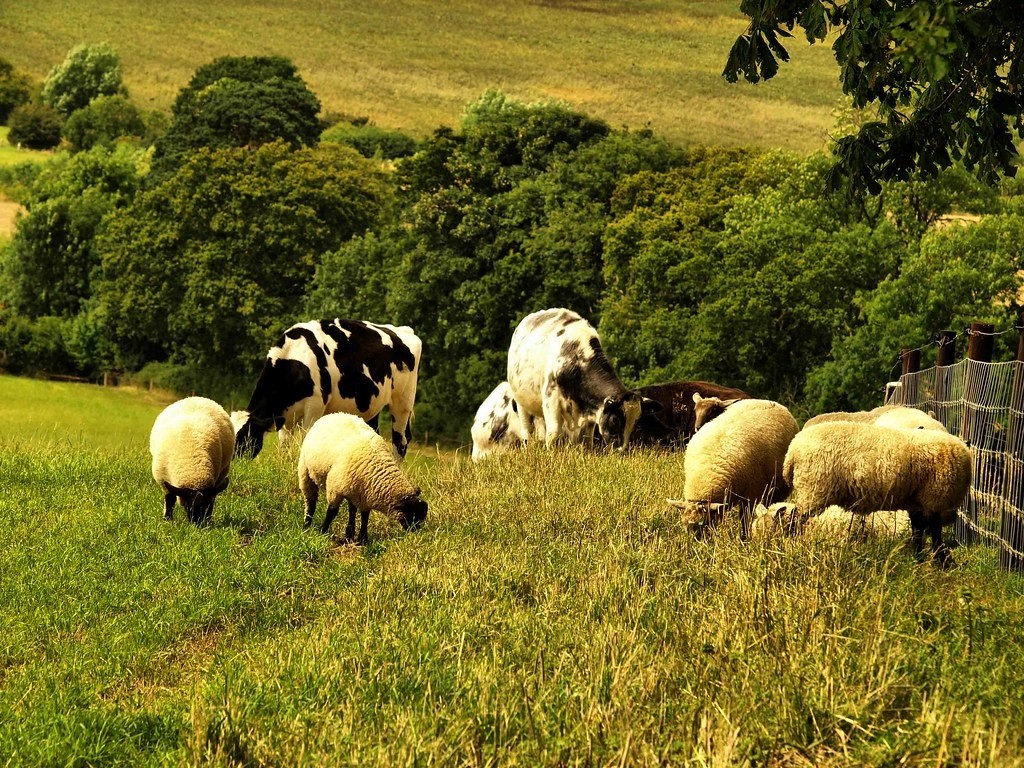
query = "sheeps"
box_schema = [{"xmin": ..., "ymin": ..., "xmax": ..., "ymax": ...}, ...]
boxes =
[
  {"xmin": 296, "ymin": 411, "xmax": 429, "ymax": 545},
  {"xmin": 665, "ymin": 399, "xmax": 973, "ymax": 569},
  {"xmin": 148, "ymin": 397, "xmax": 237, "ymax": 527}
]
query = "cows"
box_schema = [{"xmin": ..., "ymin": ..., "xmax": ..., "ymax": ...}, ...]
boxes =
[
  {"xmin": 470, "ymin": 309, "xmax": 750, "ymax": 467},
  {"xmin": 233, "ymin": 318, "xmax": 423, "ymax": 464}
]
